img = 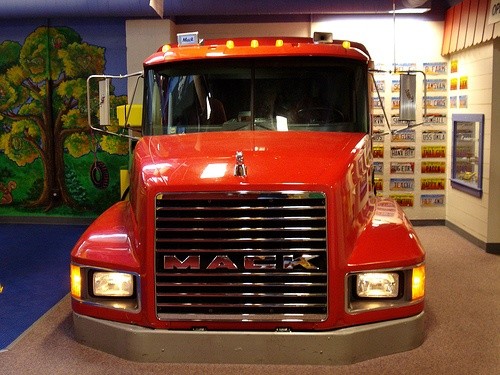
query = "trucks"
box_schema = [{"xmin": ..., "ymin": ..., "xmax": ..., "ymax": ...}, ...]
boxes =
[{"xmin": 69, "ymin": 32, "xmax": 427, "ymax": 367}]
